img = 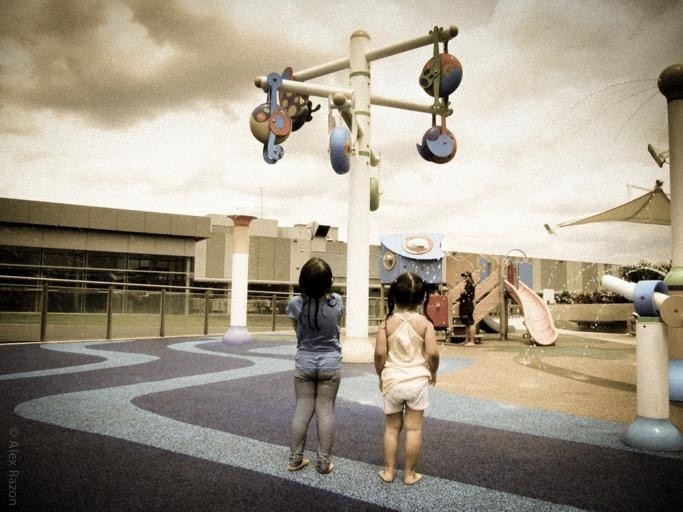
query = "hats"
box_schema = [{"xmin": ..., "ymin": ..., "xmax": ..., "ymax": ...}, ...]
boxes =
[{"xmin": 461, "ymin": 271, "xmax": 471, "ymax": 277}]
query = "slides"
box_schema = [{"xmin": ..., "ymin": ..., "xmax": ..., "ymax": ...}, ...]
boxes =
[{"xmin": 504, "ymin": 279, "xmax": 559, "ymax": 346}]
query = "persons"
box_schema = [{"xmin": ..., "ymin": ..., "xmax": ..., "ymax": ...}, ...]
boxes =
[
  {"xmin": 286, "ymin": 257, "xmax": 343, "ymax": 474},
  {"xmin": 375, "ymin": 272, "xmax": 439, "ymax": 485},
  {"xmin": 455, "ymin": 271, "xmax": 475, "ymax": 346}
]
